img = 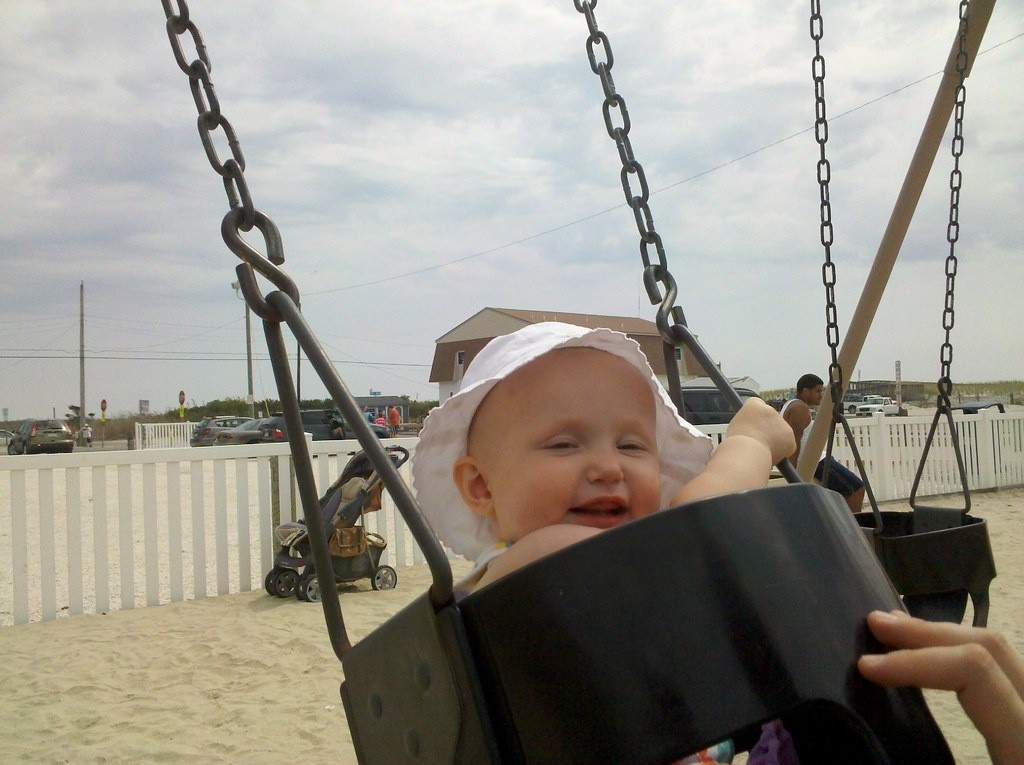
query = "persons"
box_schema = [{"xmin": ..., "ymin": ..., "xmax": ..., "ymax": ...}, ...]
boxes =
[
  {"xmin": 387, "ymin": 405, "xmax": 400, "ymax": 437},
  {"xmin": 82, "ymin": 422, "xmax": 93, "ymax": 447},
  {"xmin": 328, "ymin": 414, "xmax": 344, "ymax": 438},
  {"xmin": 411, "ymin": 318, "xmax": 798, "ymax": 765},
  {"xmin": 776, "ymin": 372, "xmax": 865, "ymax": 515},
  {"xmin": 859, "ymin": 605, "xmax": 1024, "ymax": 764},
  {"xmin": 376, "ymin": 413, "xmax": 386, "ymax": 425},
  {"xmin": 363, "ymin": 405, "xmax": 375, "ymax": 427}
]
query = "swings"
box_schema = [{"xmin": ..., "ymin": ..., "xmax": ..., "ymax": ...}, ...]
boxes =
[{"xmin": 161, "ymin": 1, "xmax": 996, "ymax": 765}]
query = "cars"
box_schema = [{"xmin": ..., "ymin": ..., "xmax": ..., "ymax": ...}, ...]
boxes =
[{"xmin": 213, "ymin": 417, "xmax": 269, "ymax": 459}]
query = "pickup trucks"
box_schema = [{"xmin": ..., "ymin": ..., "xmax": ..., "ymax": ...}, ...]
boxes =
[
  {"xmin": 856, "ymin": 397, "xmax": 899, "ymax": 416},
  {"xmin": 841, "ymin": 394, "xmax": 882, "ymax": 414}
]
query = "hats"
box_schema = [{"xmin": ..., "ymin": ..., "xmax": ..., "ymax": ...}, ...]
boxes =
[{"xmin": 416, "ymin": 321, "xmax": 716, "ymax": 565}]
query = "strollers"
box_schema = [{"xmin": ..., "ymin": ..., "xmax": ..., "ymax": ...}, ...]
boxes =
[{"xmin": 264, "ymin": 444, "xmax": 410, "ymax": 603}]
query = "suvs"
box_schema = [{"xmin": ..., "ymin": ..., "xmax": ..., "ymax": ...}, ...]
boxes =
[
  {"xmin": 657, "ymin": 377, "xmax": 769, "ymax": 445},
  {"xmin": 189, "ymin": 415, "xmax": 255, "ymax": 447},
  {"xmin": 7, "ymin": 419, "xmax": 73, "ymax": 456},
  {"xmin": 260, "ymin": 409, "xmax": 393, "ymax": 444}
]
